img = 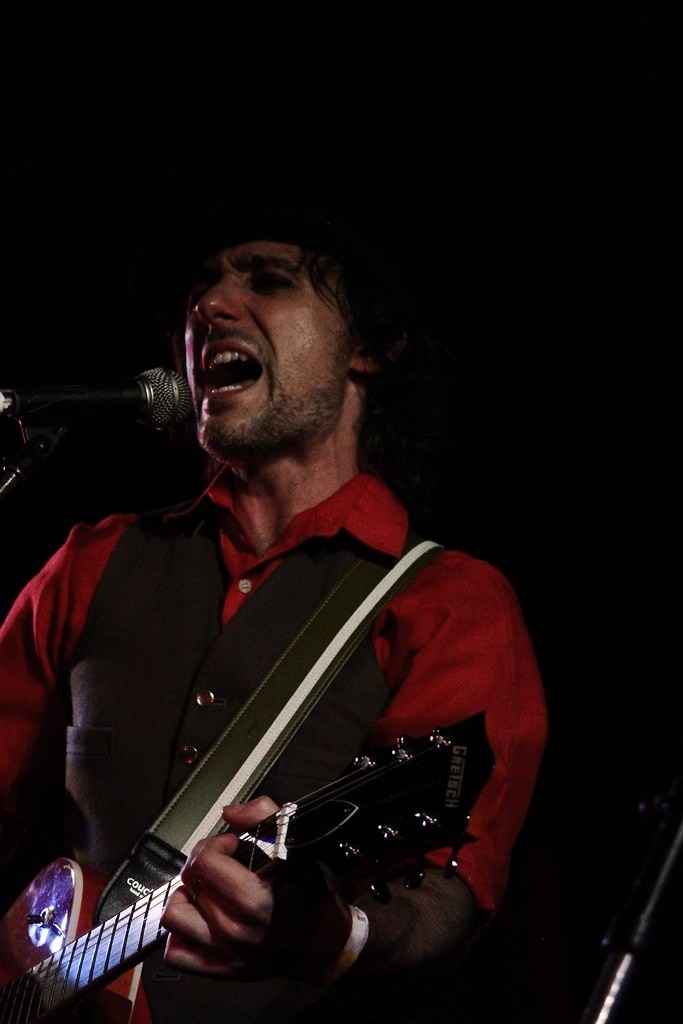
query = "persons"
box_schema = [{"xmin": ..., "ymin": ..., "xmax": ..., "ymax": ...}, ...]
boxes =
[{"xmin": 0, "ymin": 179, "xmax": 546, "ymax": 1024}]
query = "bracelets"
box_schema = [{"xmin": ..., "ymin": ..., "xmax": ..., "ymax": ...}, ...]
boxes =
[{"xmin": 314, "ymin": 896, "xmax": 373, "ymax": 994}]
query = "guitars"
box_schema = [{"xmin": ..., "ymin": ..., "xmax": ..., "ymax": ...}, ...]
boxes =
[{"xmin": 0, "ymin": 710, "xmax": 499, "ymax": 1024}]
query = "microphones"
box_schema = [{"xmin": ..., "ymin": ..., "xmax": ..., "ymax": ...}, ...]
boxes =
[{"xmin": 0, "ymin": 366, "xmax": 192, "ymax": 428}]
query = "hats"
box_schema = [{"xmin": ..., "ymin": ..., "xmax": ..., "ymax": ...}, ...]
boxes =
[{"xmin": 126, "ymin": 184, "xmax": 457, "ymax": 407}]
